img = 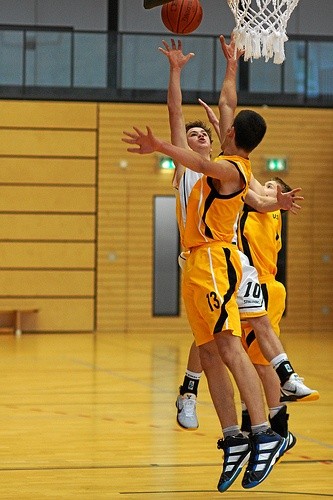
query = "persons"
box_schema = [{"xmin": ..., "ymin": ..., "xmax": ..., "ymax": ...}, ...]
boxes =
[
  {"xmin": 121, "ymin": 33, "xmax": 287, "ymax": 492},
  {"xmin": 199, "ymin": 100, "xmax": 297, "ymax": 454},
  {"xmin": 157, "ymin": 38, "xmax": 320, "ymax": 431}
]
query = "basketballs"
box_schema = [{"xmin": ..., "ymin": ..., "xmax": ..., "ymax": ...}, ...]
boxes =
[{"xmin": 161, "ymin": 0, "xmax": 203, "ymax": 34}]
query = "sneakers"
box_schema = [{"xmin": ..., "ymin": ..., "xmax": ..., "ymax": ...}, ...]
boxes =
[
  {"xmin": 241, "ymin": 430, "xmax": 289, "ymax": 489},
  {"xmin": 280, "ymin": 373, "xmax": 319, "ymax": 401},
  {"xmin": 175, "ymin": 395, "xmax": 199, "ymax": 430},
  {"xmin": 217, "ymin": 433, "xmax": 251, "ymax": 493},
  {"xmin": 283, "ymin": 432, "xmax": 297, "ymax": 452}
]
